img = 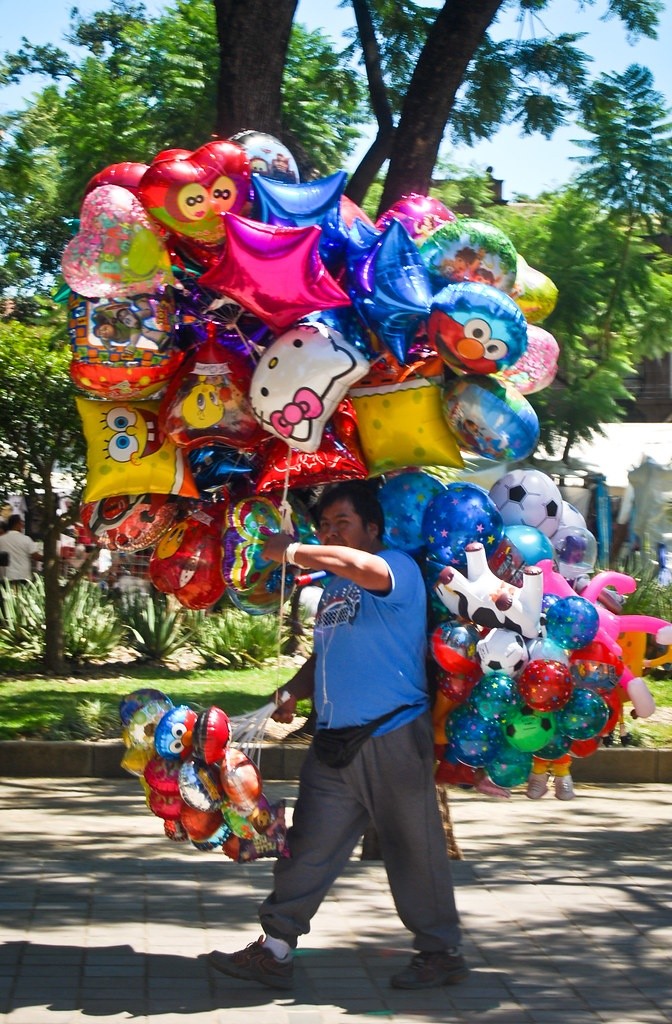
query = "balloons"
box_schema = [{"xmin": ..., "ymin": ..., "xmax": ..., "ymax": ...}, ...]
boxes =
[{"xmin": 60, "ymin": 129, "xmax": 672, "ymax": 860}]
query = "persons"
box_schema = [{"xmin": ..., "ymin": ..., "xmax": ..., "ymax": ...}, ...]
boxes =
[
  {"xmin": 206, "ymin": 480, "xmax": 470, "ymax": 990},
  {"xmin": 0, "ymin": 514, "xmax": 45, "ymax": 590}
]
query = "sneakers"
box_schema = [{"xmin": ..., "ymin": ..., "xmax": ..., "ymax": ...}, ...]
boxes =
[
  {"xmin": 527, "ymin": 774, "xmax": 575, "ymax": 802},
  {"xmin": 390, "ymin": 946, "xmax": 470, "ymax": 991},
  {"xmin": 210, "ymin": 936, "xmax": 294, "ymax": 992}
]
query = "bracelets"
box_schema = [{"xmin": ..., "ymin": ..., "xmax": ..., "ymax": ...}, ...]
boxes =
[{"xmin": 287, "ymin": 541, "xmax": 312, "ymax": 569}]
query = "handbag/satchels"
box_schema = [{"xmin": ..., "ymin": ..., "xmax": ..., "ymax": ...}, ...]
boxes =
[{"xmin": 312, "ymin": 722, "xmax": 370, "ymax": 769}]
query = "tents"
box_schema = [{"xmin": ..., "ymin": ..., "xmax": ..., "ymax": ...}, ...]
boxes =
[{"xmin": 533, "ymin": 422, "xmax": 672, "ymax": 545}]
